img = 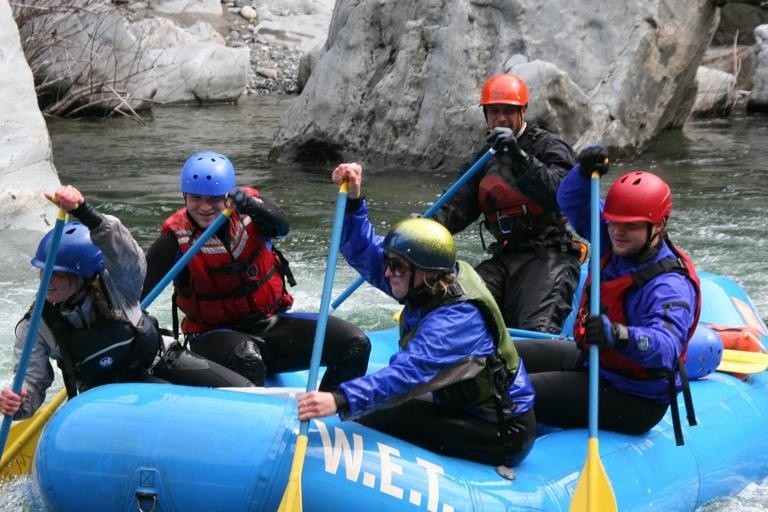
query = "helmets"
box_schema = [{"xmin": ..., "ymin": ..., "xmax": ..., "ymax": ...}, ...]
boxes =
[
  {"xmin": 601, "ymin": 171, "xmax": 671, "ymax": 224},
  {"xmin": 380, "ymin": 217, "xmax": 457, "ymax": 273},
  {"xmin": 479, "ymin": 73, "xmax": 528, "ymax": 107},
  {"xmin": 30, "ymin": 221, "xmax": 104, "ymax": 279},
  {"xmin": 180, "ymin": 151, "xmax": 236, "ymax": 197}
]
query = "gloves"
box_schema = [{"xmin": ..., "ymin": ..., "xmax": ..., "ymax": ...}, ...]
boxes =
[
  {"xmin": 582, "ymin": 313, "xmax": 628, "ymax": 351},
  {"xmin": 578, "ymin": 146, "xmax": 609, "ymax": 180},
  {"xmin": 484, "ymin": 126, "xmax": 528, "ymax": 163},
  {"xmin": 226, "ymin": 188, "xmax": 258, "ymax": 214}
]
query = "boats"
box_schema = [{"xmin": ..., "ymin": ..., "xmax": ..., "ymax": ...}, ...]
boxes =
[{"xmin": 41, "ymin": 270, "xmax": 766, "ymax": 510}]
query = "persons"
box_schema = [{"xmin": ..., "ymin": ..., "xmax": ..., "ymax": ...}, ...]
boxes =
[
  {"xmin": 500, "ymin": 141, "xmax": 702, "ymax": 437},
  {"xmin": 143, "ymin": 149, "xmax": 370, "ymax": 394},
  {"xmin": 296, "ymin": 158, "xmax": 538, "ymax": 469},
  {"xmin": 422, "ymin": 71, "xmax": 585, "ymax": 338},
  {"xmin": 0, "ymin": 181, "xmax": 261, "ymax": 422}
]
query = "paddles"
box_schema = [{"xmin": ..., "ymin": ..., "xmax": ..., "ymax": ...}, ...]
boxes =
[
  {"xmin": 501, "ymin": 326, "xmax": 767, "ymax": 376},
  {"xmin": 569, "ymin": 168, "xmax": 617, "ymax": 512},
  {"xmin": 1, "ymin": 207, "xmax": 230, "ymax": 485},
  {"xmin": 276, "ymin": 176, "xmax": 347, "ymax": 512}
]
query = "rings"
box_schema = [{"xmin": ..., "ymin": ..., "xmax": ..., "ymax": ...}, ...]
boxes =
[{"xmin": 302, "ymin": 399, "xmax": 309, "ymax": 406}]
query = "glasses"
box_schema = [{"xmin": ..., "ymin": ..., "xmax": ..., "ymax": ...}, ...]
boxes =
[{"xmin": 386, "ymin": 256, "xmax": 411, "ymax": 278}]
